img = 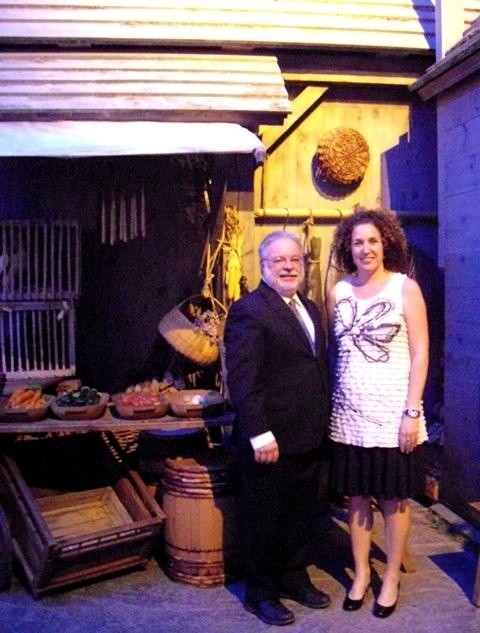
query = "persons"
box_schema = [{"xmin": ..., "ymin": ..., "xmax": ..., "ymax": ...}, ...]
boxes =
[
  {"xmin": 218, "ymin": 229, "xmax": 333, "ymax": 625},
  {"xmin": 323, "ymin": 206, "xmax": 431, "ymax": 619}
]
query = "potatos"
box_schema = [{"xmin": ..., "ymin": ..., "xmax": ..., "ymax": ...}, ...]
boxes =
[{"xmin": 120, "ymin": 393, "xmax": 160, "ymax": 407}]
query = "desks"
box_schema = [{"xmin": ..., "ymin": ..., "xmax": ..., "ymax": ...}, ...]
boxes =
[{"xmin": 2, "ymin": 391, "xmax": 236, "ymax": 438}]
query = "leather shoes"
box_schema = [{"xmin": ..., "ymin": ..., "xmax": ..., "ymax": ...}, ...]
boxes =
[
  {"xmin": 280, "ymin": 585, "xmax": 330, "ymax": 608},
  {"xmin": 243, "ymin": 599, "xmax": 295, "ymax": 625},
  {"xmin": 342, "ymin": 589, "xmax": 363, "ymax": 611},
  {"xmin": 373, "ymin": 581, "xmax": 400, "ymax": 618}
]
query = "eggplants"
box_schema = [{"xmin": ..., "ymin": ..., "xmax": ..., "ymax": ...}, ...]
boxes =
[{"xmin": 56, "ymin": 386, "xmax": 101, "ymax": 407}]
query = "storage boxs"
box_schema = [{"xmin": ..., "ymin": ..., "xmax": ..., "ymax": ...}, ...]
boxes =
[{"xmin": 0, "ymin": 431, "xmax": 172, "ymax": 599}]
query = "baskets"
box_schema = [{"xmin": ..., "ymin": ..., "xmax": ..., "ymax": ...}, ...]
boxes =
[
  {"xmin": 316, "ymin": 126, "xmax": 370, "ymax": 187},
  {"xmin": 157, "ymin": 295, "xmax": 228, "ymax": 368}
]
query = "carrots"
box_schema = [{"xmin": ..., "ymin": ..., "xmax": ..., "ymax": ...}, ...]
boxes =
[{"xmin": 9, "ymin": 389, "xmax": 46, "ymax": 409}]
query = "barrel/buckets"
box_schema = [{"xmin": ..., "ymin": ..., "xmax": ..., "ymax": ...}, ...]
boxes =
[{"xmin": 159, "ymin": 452, "xmax": 241, "ymax": 587}]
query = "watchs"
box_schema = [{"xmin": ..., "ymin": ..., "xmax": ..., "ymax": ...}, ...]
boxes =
[{"xmin": 401, "ymin": 408, "xmax": 422, "ymax": 419}]
